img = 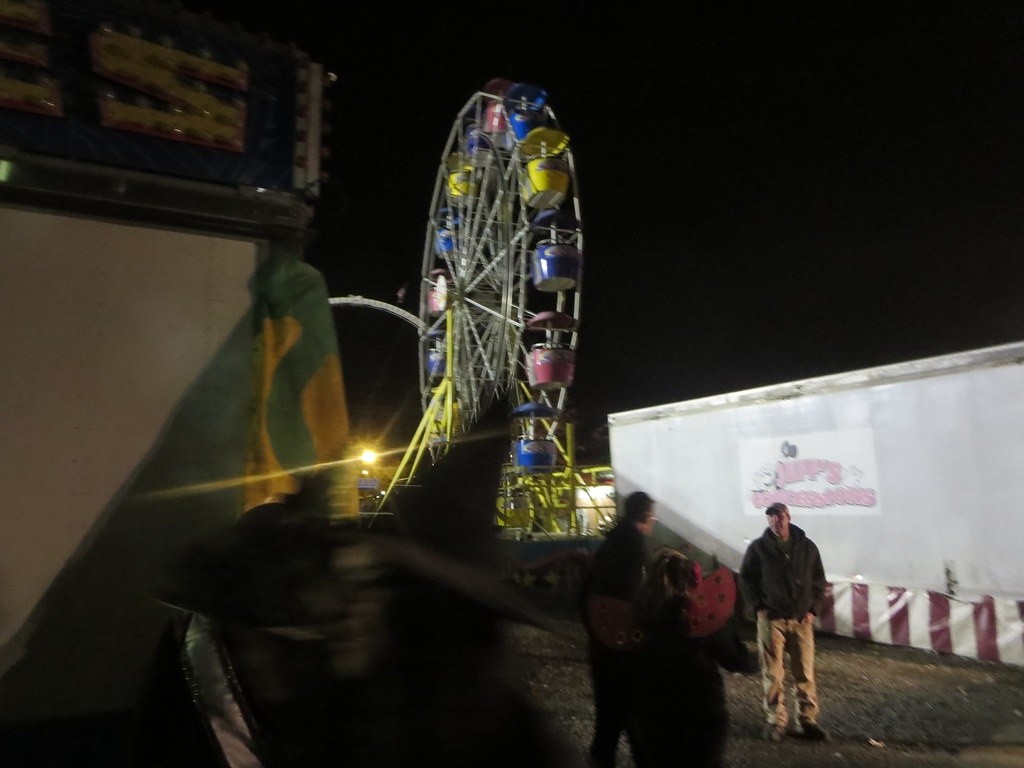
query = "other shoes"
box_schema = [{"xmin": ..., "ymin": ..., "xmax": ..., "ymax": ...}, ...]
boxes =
[
  {"xmin": 795, "ymin": 721, "xmax": 828, "ymax": 740},
  {"xmin": 762, "ymin": 721, "xmax": 786, "ymax": 743}
]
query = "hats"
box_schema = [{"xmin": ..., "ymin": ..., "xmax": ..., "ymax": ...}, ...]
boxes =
[{"xmin": 766, "ymin": 502, "xmax": 786, "ymax": 514}]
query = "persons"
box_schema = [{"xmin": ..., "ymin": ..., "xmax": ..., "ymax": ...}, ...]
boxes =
[
  {"xmin": 582, "ymin": 491, "xmax": 759, "ymax": 768},
  {"xmin": 739, "ymin": 502, "xmax": 829, "ymax": 741}
]
